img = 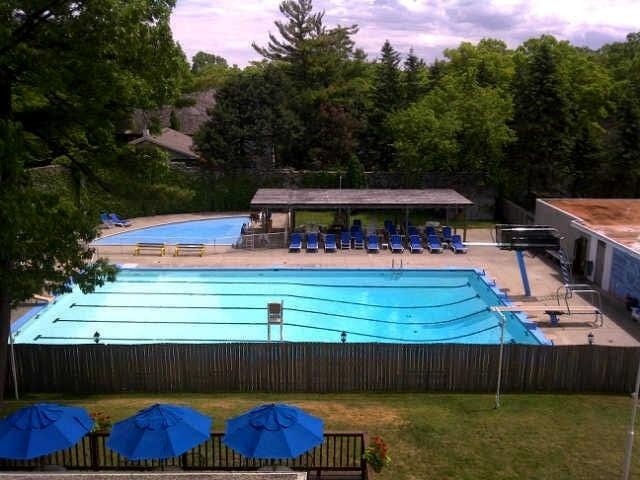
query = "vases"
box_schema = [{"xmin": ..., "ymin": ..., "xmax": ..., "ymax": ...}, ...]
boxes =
[{"xmin": 370, "ymin": 462, "xmax": 385, "ymax": 473}]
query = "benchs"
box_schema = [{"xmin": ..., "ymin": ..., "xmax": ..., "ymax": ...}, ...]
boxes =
[
  {"xmin": 175, "ymin": 243, "xmax": 205, "ymax": 257},
  {"xmin": 134, "ymin": 242, "xmax": 167, "ymax": 257}
]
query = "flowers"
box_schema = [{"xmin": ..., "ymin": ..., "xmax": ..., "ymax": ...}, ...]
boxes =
[{"xmin": 364, "ymin": 436, "xmax": 392, "ymax": 465}]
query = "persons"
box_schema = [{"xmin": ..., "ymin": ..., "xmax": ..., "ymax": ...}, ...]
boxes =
[{"xmin": 241, "ymin": 223, "xmax": 246, "ymax": 247}]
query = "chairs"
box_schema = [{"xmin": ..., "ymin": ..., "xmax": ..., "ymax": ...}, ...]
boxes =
[
  {"xmin": 289, "ymin": 219, "xmax": 469, "ymax": 255},
  {"xmin": 100, "ymin": 213, "xmax": 116, "ymax": 229},
  {"xmin": 110, "ymin": 213, "xmax": 133, "ymax": 227}
]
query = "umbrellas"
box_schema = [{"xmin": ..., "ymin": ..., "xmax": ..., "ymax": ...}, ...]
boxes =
[
  {"xmin": 0, "ymin": 403, "xmax": 94, "ymax": 466},
  {"xmin": 105, "ymin": 403, "xmax": 213, "ymax": 471},
  {"xmin": 222, "ymin": 403, "xmax": 324, "ymax": 470}
]
